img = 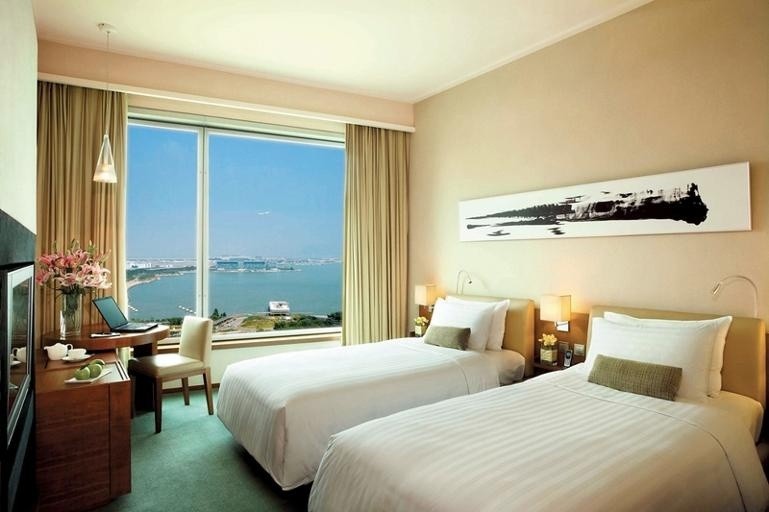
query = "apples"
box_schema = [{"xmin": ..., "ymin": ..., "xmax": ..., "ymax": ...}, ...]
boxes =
[
  {"xmin": 75, "ymin": 366, "xmax": 90, "ymax": 380},
  {"xmin": 88, "ymin": 364, "xmax": 102, "ymax": 378},
  {"xmin": 91, "ymin": 359, "xmax": 104, "ymax": 369}
]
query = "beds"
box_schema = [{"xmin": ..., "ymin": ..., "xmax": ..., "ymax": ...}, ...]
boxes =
[
  {"xmin": 304, "ymin": 307, "xmax": 768, "ymax": 512},
  {"xmin": 216, "ymin": 292, "xmax": 534, "ymax": 492}
]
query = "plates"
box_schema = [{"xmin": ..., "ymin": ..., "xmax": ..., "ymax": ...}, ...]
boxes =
[
  {"xmin": 60, "ymin": 354, "xmax": 92, "ymax": 363},
  {"xmin": 9, "ymin": 361, "xmax": 20, "ymax": 367}
]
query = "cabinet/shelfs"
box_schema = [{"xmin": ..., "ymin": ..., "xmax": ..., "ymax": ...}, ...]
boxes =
[{"xmin": 34, "ymin": 350, "xmax": 132, "ymax": 511}]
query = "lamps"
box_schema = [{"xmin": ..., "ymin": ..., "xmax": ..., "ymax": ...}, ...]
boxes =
[
  {"xmin": 456, "ymin": 270, "xmax": 473, "ymax": 294},
  {"xmin": 415, "ymin": 285, "xmax": 436, "ymax": 313},
  {"xmin": 539, "ymin": 293, "xmax": 572, "ymax": 332},
  {"xmin": 711, "ymin": 275, "xmax": 759, "ymax": 318},
  {"xmin": 92, "ymin": 23, "xmax": 119, "ymax": 183}
]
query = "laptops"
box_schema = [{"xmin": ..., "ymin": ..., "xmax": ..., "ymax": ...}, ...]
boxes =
[{"xmin": 91, "ymin": 296, "xmax": 159, "ymax": 332}]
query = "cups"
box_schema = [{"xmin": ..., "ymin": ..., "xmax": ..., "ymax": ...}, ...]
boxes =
[{"xmin": 67, "ymin": 347, "xmax": 87, "ymax": 359}]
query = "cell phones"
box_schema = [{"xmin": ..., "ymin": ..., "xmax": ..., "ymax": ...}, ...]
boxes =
[{"xmin": 563, "ymin": 350, "xmax": 573, "ymax": 367}]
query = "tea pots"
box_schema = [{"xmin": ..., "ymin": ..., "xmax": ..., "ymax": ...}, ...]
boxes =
[
  {"xmin": 11, "ymin": 347, "xmax": 27, "ymax": 364},
  {"xmin": 43, "ymin": 342, "xmax": 73, "ymax": 361}
]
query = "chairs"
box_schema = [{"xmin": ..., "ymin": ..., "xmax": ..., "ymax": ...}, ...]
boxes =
[{"xmin": 126, "ymin": 315, "xmax": 213, "ymax": 433}]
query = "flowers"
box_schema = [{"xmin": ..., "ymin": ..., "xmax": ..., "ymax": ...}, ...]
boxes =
[
  {"xmin": 36, "ymin": 238, "xmax": 114, "ymax": 328},
  {"xmin": 413, "ymin": 317, "xmax": 429, "ymax": 325},
  {"xmin": 537, "ymin": 332, "xmax": 557, "ymax": 348}
]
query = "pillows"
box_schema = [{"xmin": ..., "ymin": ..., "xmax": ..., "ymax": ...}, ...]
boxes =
[
  {"xmin": 585, "ymin": 318, "xmax": 719, "ymax": 403},
  {"xmin": 587, "ymin": 354, "xmax": 682, "ymax": 402},
  {"xmin": 429, "ymin": 297, "xmax": 497, "ymax": 351},
  {"xmin": 604, "ymin": 311, "xmax": 732, "ymax": 398},
  {"xmin": 445, "ymin": 295, "xmax": 509, "ymax": 351},
  {"xmin": 424, "ymin": 325, "xmax": 471, "ymax": 351}
]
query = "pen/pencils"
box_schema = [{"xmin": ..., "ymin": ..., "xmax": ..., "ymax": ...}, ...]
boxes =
[{"xmin": 97, "ymin": 333, "xmax": 110, "ymax": 334}]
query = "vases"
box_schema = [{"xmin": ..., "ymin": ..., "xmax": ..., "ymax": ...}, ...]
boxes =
[
  {"xmin": 60, "ymin": 292, "xmax": 83, "ymax": 337},
  {"xmin": 414, "ymin": 323, "xmax": 428, "ymax": 337},
  {"xmin": 539, "ymin": 343, "xmax": 557, "ymax": 367}
]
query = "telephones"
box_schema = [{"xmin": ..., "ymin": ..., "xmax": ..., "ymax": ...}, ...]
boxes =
[{"xmin": 561, "ymin": 349, "xmax": 573, "ymax": 370}]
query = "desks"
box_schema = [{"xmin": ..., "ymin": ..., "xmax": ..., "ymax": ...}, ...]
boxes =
[{"xmin": 42, "ymin": 320, "xmax": 170, "ymax": 417}]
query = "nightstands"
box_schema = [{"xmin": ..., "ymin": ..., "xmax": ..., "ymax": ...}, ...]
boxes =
[{"xmin": 534, "ymin": 356, "xmax": 578, "ymax": 376}]
